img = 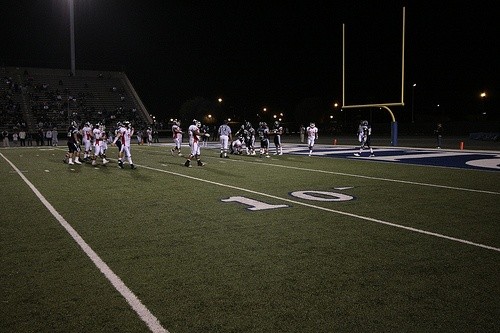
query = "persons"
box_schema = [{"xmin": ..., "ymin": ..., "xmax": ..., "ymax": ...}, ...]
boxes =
[
  {"xmin": 137, "ymin": 126, "xmax": 159, "ymax": 145},
  {"xmin": 63, "ymin": 120, "xmax": 137, "ymax": 169},
  {"xmin": 333, "ymin": 128, "xmax": 336, "ymax": 145},
  {"xmin": 2, "ymin": 126, "xmax": 58, "ymax": 147},
  {"xmin": 202, "ymin": 126, "xmax": 209, "ymax": 146},
  {"xmin": 0, "ymin": 63, "xmax": 147, "ymax": 129},
  {"xmin": 257, "ymin": 120, "xmax": 283, "ymax": 157},
  {"xmin": 171, "ymin": 119, "xmax": 184, "ymax": 156},
  {"xmin": 357, "ymin": 120, "xmax": 363, "ymax": 136},
  {"xmin": 436, "ymin": 123, "xmax": 442, "ymax": 147},
  {"xmin": 300, "ymin": 124, "xmax": 305, "ymax": 143},
  {"xmin": 219, "ymin": 120, "xmax": 256, "ymax": 158},
  {"xmin": 306, "ymin": 122, "xmax": 318, "ymax": 156},
  {"xmin": 354, "ymin": 120, "xmax": 374, "ymax": 157},
  {"xmin": 185, "ymin": 120, "xmax": 205, "ymax": 168}
]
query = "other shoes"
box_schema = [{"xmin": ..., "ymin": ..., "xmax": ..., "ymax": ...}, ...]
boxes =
[
  {"xmin": 230, "ymin": 148, "xmax": 283, "ymax": 158},
  {"xmin": 68, "ymin": 156, "xmax": 92, "ymax": 164},
  {"xmin": 103, "ymin": 160, "xmax": 110, "ymax": 164},
  {"xmin": 92, "ymin": 162, "xmax": 97, "ymax": 165},
  {"xmin": 170, "ymin": 149, "xmax": 174, "ymax": 155},
  {"xmin": 308, "ymin": 152, "xmax": 312, "ymax": 156},
  {"xmin": 131, "ymin": 166, "xmax": 136, "ymax": 169},
  {"xmin": 197, "ymin": 163, "xmax": 205, "ymax": 166},
  {"xmin": 178, "ymin": 152, "xmax": 182, "ymax": 156},
  {"xmin": 185, "ymin": 163, "xmax": 192, "ymax": 167},
  {"xmin": 118, "ymin": 159, "xmax": 121, "ymax": 163},
  {"xmin": 219, "ymin": 152, "xmax": 229, "ymax": 158},
  {"xmin": 354, "ymin": 152, "xmax": 375, "ymax": 157},
  {"xmin": 118, "ymin": 166, "xmax": 124, "ymax": 169}
]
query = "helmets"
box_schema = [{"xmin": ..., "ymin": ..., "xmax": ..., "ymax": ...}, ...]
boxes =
[
  {"xmin": 71, "ymin": 121, "xmax": 77, "ymax": 128},
  {"xmin": 259, "ymin": 122, "xmax": 263, "ymax": 126},
  {"xmin": 198, "ymin": 121, "xmax": 202, "ymax": 128},
  {"xmin": 122, "ymin": 121, "xmax": 131, "ymax": 128},
  {"xmin": 96, "ymin": 122, "xmax": 102, "ymax": 128},
  {"xmin": 85, "ymin": 121, "xmax": 90, "ymax": 127},
  {"xmin": 173, "ymin": 119, "xmax": 180, "ymax": 126},
  {"xmin": 274, "ymin": 121, "xmax": 279, "ymax": 128},
  {"xmin": 192, "ymin": 119, "xmax": 198, "ymax": 125},
  {"xmin": 310, "ymin": 123, "xmax": 315, "ymax": 128},
  {"xmin": 117, "ymin": 121, "xmax": 123, "ymax": 129},
  {"xmin": 363, "ymin": 121, "xmax": 368, "ymax": 125}
]
